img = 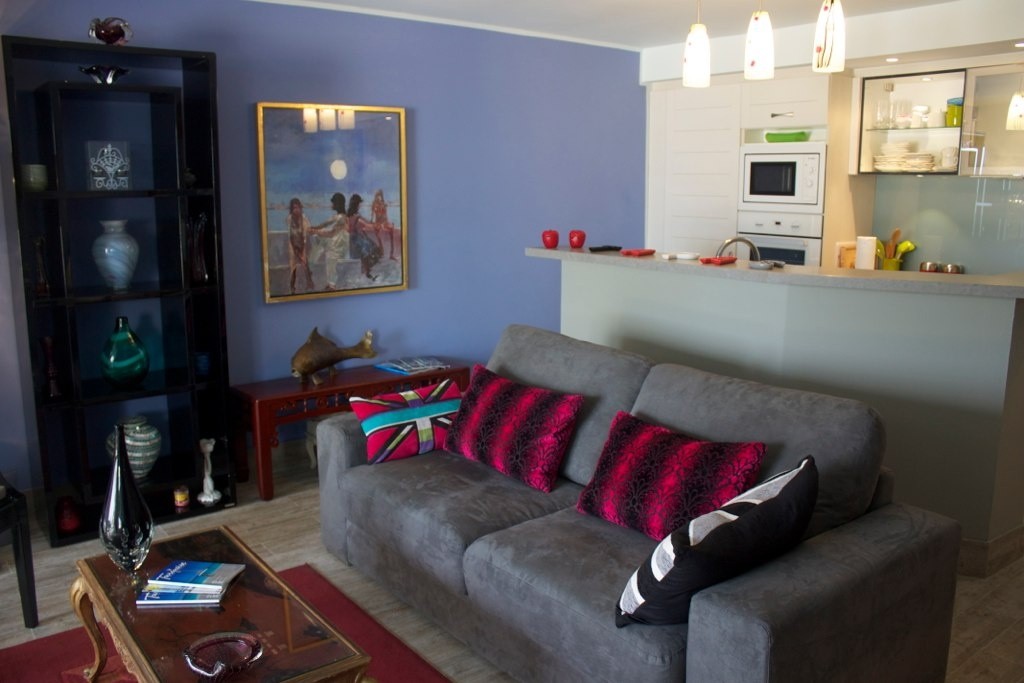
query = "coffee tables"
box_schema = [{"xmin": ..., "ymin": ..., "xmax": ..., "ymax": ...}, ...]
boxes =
[
  {"xmin": 231, "ymin": 360, "xmax": 470, "ymax": 502},
  {"xmin": 71, "ymin": 524, "xmax": 377, "ymax": 683}
]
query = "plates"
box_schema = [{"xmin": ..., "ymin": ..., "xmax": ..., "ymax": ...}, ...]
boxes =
[
  {"xmin": 932, "ymin": 166, "xmax": 957, "ymax": 172},
  {"xmin": 873, "ymin": 141, "xmax": 934, "ymax": 172}
]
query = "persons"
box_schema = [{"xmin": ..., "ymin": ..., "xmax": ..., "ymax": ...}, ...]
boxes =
[{"xmin": 284, "ymin": 190, "xmax": 402, "ymax": 295}]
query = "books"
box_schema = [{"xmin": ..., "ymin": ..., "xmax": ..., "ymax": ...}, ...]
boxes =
[{"xmin": 135, "ymin": 559, "xmax": 247, "ymax": 607}]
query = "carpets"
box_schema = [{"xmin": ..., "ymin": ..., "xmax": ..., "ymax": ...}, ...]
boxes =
[{"xmin": 0, "ymin": 562, "xmax": 454, "ymax": 683}]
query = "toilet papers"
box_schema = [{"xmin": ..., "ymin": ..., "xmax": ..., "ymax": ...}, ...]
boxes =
[{"xmin": 854, "ymin": 235, "xmax": 877, "ymax": 270}]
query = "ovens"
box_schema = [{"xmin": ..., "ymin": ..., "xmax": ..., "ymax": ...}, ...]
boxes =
[{"xmin": 736, "ymin": 210, "xmax": 823, "ymax": 268}]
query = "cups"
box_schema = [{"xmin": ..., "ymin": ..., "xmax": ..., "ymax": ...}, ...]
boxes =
[
  {"xmin": 892, "ymin": 100, "xmax": 911, "ymax": 128},
  {"xmin": 194, "ymin": 352, "xmax": 210, "ymax": 376},
  {"xmin": 873, "ymin": 99, "xmax": 893, "ymax": 129},
  {"xmin": 941, "ymin": 146, "xmax": 958, "ymax": 167},
  {"xmin": 880, "ymin": 259, "xmax": 903, "ymax": 271}
]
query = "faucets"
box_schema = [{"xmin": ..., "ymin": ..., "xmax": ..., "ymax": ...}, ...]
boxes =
[{"xmin": 714, "ymin": 236, "xmax": 761, "ymax": 261}]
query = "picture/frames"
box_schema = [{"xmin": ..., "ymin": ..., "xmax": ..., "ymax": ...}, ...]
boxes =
[{"xmin": 256, "ymin": 101, "xmax": 409, "ymax": 303}]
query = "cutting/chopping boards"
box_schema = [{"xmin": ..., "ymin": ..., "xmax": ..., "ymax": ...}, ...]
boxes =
[{"xmin": 831, "ymin": 241, "xmax": 856, "ymax": 269}]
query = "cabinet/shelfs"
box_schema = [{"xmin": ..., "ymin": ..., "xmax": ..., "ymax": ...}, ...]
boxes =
[
  {"xmin": 1, "ymin": 35, "xmax": 237, "ymax": 549},
  {"xmin": 643, "ymin": 53, "xmax": 1024, "ymax": 258}
]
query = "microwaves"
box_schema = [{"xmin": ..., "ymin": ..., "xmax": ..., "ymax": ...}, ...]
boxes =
[{"xmin": 743, "ymin": 154, "xmax": 819, "ymax": 204}]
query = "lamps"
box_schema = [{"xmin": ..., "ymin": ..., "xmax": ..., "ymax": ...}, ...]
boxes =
[
  {"xmin": 812, "ymin": 0, "xmax": 846, "ymax": 73},
  {"xmin": 682, "ymin": 0, "xmax": 712, "ymax": 89},
  {"xmin": 744, "ymin": 0, "xmax": 774, "ymax": 81}
]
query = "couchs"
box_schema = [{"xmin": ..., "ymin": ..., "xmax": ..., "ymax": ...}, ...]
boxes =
[{"xmin": 316, "ymin": 324, "xmax": 964, "ymax": 683}]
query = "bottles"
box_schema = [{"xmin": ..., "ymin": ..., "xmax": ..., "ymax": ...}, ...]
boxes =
[
  {"xmin": 90, "ymin": 218, "xmax": 140, "ymax": 292},
  {"xmin": 919, "ymin": 262, "xmax": 959, "ymax": 274},
  {"xmin": 100, "ymin": 316, "xmax": 149, "ymax": 385},
  {"xmin": 911, "ymin": 97, "xmax": 963, "ymax": 128},
  {"xmin": 18, "ymin": 131, "xmax": 48, "ymax": 193},
  {"xmin": 97, "ymin": 415, "xmax": 161, "ymax": 572}
]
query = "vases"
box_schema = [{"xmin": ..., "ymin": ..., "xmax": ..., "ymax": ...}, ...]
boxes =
[
  {"xmin": 104, "ymin": 415, "xmax": 162, "ymax": 484},
  {"xmin": 98, "ymin": 421, "xmax": 154, "ymax": 572},
  {"xmin": 90, "ymin": 218, "xmax": 139, "ymax": 290},
  {"xmin": 20, "ymin": 163, "xmax": 49, "ymax": 194}
]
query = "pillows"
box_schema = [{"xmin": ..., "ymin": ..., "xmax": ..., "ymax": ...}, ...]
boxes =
[
  {"xmin": 347, "ymin": 378, "xmax": 466, "ymax": 466},
  {"xmin": 614, "ymin": 455, "xmax": 820, "ymax": 628},
  {"xmin": 441, "ymin": 362, "xmax": 585, "ymax": 496},
  {"xmin": 577, "ymin": 410, "xmax": 769, "ymax": 542}
]
